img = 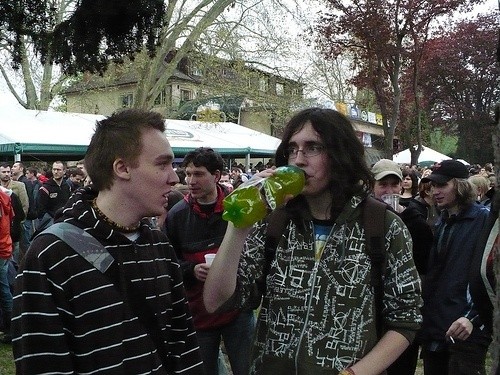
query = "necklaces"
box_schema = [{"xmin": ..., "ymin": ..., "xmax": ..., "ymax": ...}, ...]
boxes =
[{"xmin": 91, "ymin": 197, "xmax": 141, "ymax": 233}]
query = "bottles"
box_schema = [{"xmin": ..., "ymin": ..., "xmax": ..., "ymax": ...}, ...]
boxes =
[{"xmin": 221, "ymin": 165, "xmax": 307, "ymax": 229}]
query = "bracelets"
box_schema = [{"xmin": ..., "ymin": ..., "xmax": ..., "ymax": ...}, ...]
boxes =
[{"xmin": 338, "ymin": 368, "xmax": 355, "ymax": 375}]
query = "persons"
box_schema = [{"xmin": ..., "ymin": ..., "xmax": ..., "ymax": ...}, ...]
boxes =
[
  {"xmin": 359, "ymin": 159, "xmax": 500, "ymax": 375},
  {"xmin": 203, "ymin": 108, "xmax": 423, "ymax": 375},
  {"xmin": 10, "ymin": 109, "xmax": 204, "ymax": 375},
  {"xmin": 142, "ymin": 147, "xmax": 275, "ymax": 375},
  {"xmin": 0, "ymin": 158, "xmax": 93, "ymax": 343}
]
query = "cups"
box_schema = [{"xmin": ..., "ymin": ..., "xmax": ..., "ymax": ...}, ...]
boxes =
[
  {"xmin": 381, "ymin": 193, "xmax": 400, "ymax": 213},
  {"xmin": 204, "ymin": 254, "xmax": 216, "ymax": 265}
]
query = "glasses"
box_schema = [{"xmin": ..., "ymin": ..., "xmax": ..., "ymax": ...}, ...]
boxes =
[{"xmin": 282, "ymin": 145, "xmax": 327, "ymax": 159}]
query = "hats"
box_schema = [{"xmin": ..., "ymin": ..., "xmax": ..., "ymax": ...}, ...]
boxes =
[
  {"xmin": 420, "ymin": 159, "xmax": 469, "ymax": 185},
  {"xmin": 370, "ymin": 159, "xmax": 403, "ymax": 182}
]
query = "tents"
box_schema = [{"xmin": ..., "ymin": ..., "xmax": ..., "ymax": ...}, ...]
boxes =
[
  {"xmin": 393, "ymin": 144, "xmax": 470, "ymax": 169},
  {"xmin": 0, "ymin": 110, "xmax": 282, "ymax": 173}
]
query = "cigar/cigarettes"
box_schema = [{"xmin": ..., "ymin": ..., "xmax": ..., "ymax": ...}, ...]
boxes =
[{"xmin": 450, "ymin": 336, "xmax": 455, "ymax": 343}]
